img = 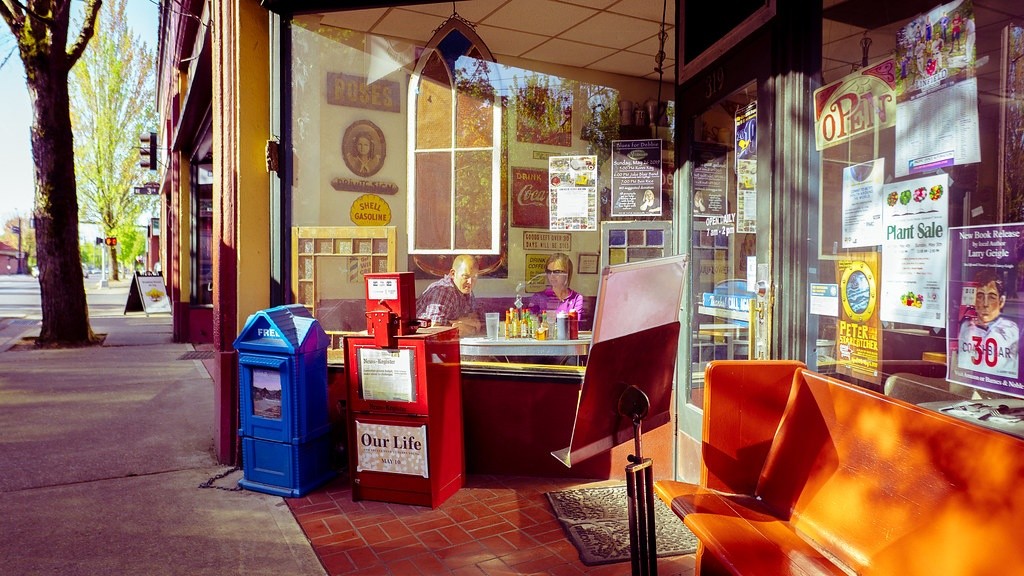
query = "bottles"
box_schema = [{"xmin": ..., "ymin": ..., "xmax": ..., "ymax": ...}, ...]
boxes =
[
  {"xmin": 520, "ymin": 309, "xmax": 532, "ymax": 338},
  {"xmin": 510, "ymin": 308, "xmax": 520, "ymax": 337},
  {"xmin": 505, "ymin": 311, "xmax": 511, "ymax": 340},
  {"xmin": 532, "ymin": 310, "xmax": 549, "ymax": 341},
  {"xmin": 568, "ymin": 309, "xmax": 579, "ymax": 338}
]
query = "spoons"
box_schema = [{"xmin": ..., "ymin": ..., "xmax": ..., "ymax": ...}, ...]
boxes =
[{"xmin": 978, "ymin": 404, "xmax": 1009, "ymax": 420}]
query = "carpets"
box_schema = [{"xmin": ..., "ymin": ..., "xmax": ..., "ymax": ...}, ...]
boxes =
[{"xmin": 545, "ymin": 483, "xmax": 699, "ymax": 565}]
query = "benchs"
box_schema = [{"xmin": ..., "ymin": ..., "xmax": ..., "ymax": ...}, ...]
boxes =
[{"xmin": 655, "ymin": 367, "xmax": 1024, "ymax": 576}]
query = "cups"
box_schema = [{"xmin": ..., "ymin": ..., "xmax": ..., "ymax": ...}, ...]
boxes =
[
  {"xmin": 547, "ymin": 311, "xmax": 555, "ymax": 337},
  {"xmin": 485, "ymin": 313, "xmax": 499, "ymax": 340},
  {"xmin": 556, "ymin": 310, "xmax": 569, "ymax": 339}
]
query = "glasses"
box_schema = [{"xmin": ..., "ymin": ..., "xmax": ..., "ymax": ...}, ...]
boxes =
[{"xmin": 545, "ymin": 268, "xmax": 567, "ymax": 275}]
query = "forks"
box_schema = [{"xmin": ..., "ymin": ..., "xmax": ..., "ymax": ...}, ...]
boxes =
[
  {"xmin": 950, "ymin": 404, "xmax": 993, "ymax": 413},
  {"xmin": 989, "ymin": 409, "xmax": 1024, "ymax": 420}
]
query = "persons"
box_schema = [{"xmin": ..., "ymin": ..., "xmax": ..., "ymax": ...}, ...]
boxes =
[
  {"xmin": 638, "ymin": 190, "xmax": 706, "ymax": 217},
  {"xmin": 348, "ymin": 129, "xmax": 380, "ymax": 173},
  {"xmin": 416, "ymin": 255, "xmax": 482, "ymax": 337},
  {"xmin": 154, "ymin": 260, "xmax": 161, "ymax": 276},
  {"xmin": 528, "ymin": 253, "xmax": 591, "ymax": 334},
  {"xmin": 899, "ymin": 10, "xmax": 976, "ymax": 93}
]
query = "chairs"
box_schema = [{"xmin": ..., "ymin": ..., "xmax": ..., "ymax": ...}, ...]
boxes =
[{"xmin": 884, "ymin": 372, "xmax": 1004, "ymax": 405}]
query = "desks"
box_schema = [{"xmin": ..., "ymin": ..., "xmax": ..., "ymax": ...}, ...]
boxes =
[
  {"xmin": 916, "ymin": 399, "xmax": 1024, "ymax": 439},
  {"xmin": 459, "ymin": 331, "xmax": 593, "ymax": 366}
]
